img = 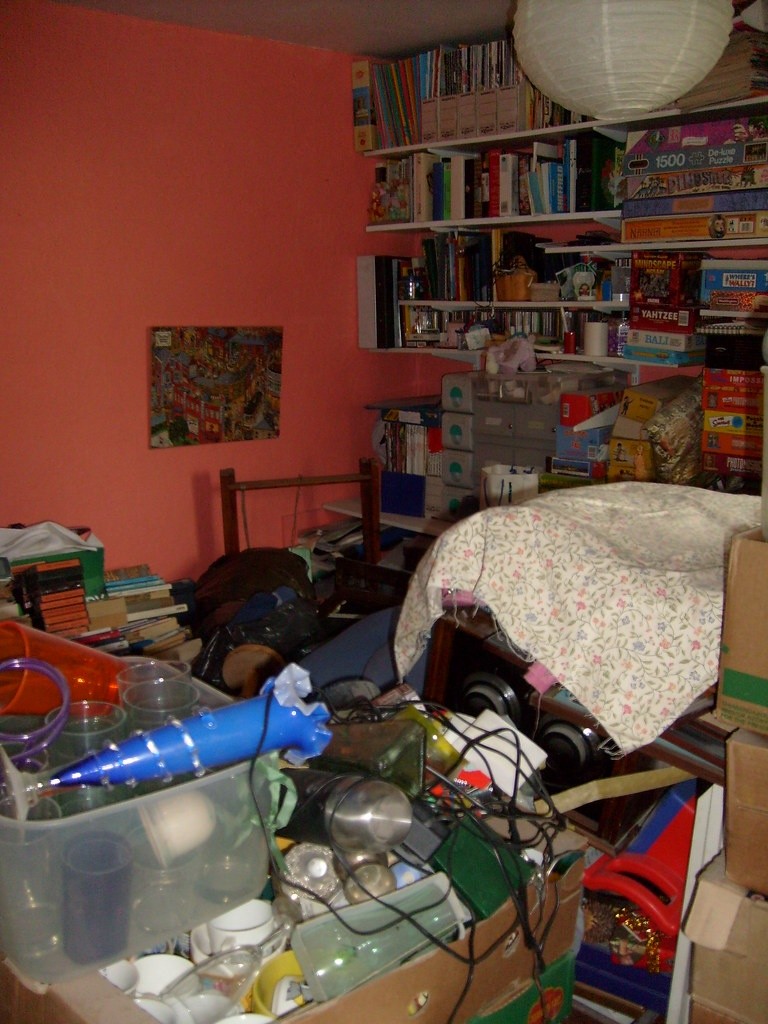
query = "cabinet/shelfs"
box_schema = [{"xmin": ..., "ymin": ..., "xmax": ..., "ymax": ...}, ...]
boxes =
[{"xmin": 366, "ymin": 96, "xmax": 768, "ymax": 368}]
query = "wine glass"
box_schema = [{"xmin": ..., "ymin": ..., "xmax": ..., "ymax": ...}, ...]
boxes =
[{"xmin": 159, "ymin": 895, "xmax": 303, "ymax": 1024}]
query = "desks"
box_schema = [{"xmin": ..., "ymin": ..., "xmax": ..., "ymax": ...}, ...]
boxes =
[{"xmin": 323, "ymin": 493, "xmax": 457, "ymax": 536}]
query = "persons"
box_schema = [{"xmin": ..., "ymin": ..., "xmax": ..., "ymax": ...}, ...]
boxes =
[
  {"xmin": 614, "ymin": 443, "xmax": 628, "ymax": 461},
  {"xmin": 621, "ymin": 397, "xmax": 633, "ymax": 415}
]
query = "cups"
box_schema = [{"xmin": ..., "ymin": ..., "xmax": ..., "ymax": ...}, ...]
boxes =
[
  {"xmin": 0, "ymin": 661, "xmax": 201, "ymax": 819},
  {"xmin": 207, "ymin": 900, "xmax": 275, "ymax": 954}
]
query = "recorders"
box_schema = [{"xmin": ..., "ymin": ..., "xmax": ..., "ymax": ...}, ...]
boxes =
[{"xmin": 421, "ymin": 602, "xmax": 675, "ymax": 847}]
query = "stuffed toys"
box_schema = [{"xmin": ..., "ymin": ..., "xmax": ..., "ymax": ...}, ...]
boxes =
[{"xmin": 487, "ymin": 335, "xmax": 537, "ymax": 374}]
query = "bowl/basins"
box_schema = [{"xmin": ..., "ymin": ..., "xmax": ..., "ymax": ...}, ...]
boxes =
[{"xmin": 98, "ymin": 898, "xmax": 314, "ymax": 1024}]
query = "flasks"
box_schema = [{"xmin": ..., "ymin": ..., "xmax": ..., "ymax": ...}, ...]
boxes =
[{"xmin": 272, "ymin": 767, "xmax": 413, "ymax": 854}]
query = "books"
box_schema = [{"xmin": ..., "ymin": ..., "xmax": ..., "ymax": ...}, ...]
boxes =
[
  {"xmin": 353, "ymin": 37, "xmax": 768, "ymax": 368},
  {"xmin": 12, "ymin": 561, "xmax": 203, "ymax": 665},
  {"xmin": 382, "ymin": 409, "xmax": 442, "ymax": 475}
]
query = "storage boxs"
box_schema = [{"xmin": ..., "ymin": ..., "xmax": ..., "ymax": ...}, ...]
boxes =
[
  {"xmin": 725, "ymin": 729, "xmax": 768, "ymax": 896},
  {"xmin": 310, "ymin": 719, "xmax": 426, "ymax": 801},
  {"xmin": 0, "ymin": 814, "xmax": 591, "ymax": 1023},
  {"xmin": 624, "ymin": 329, "xmax": 706, "ymax": 364},
  {"xmin": 381, "ymin": 472, "xmax": 479, "ymax": 522},
  {"xmin": 630, "ymin": 306, "xmax": 725, "ymax": 334},
  {"xmin": 717, "ymin": 526, "xmax": 768, "ymax": 737},
  {"xmin": 0, "ymin": 656, "xmax": 281, "ymax": 983},
  {"xmin": 684, "ymin": 849, "xmax": 768, "ymax": 1024},
  {"xmin": 698, "ymin": 260, "xmax": 768, "ymax": 313},
  {"xmin": 500, "ymin": 155, "xmax": 520, "ymax": 216},
  {"xmin": 622, "ymin": 116, "xmax": 768, "ymax": 243},
  {"xmin": 551, "ymin": 375, "xmax": 696, "ymax": 482},
  {"xmin": 701, "ymin": 369, "xmax": 764, "ymax": 479},
  {"xmin": 622, "ymin": 251, "xmax": 705, "ymax": 307},
  {"xmin": 468, "ymin": 370, "xmax": 628, "ymax": 488},
  {"xmin": 318, "ymin": 557, "xmax": 411, "ymax": 634}
]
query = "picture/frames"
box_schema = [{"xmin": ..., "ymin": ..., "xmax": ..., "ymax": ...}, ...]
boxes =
[{"xmin": 530, "ymin": 685, "xmax": 726, "ymax": 787}]
